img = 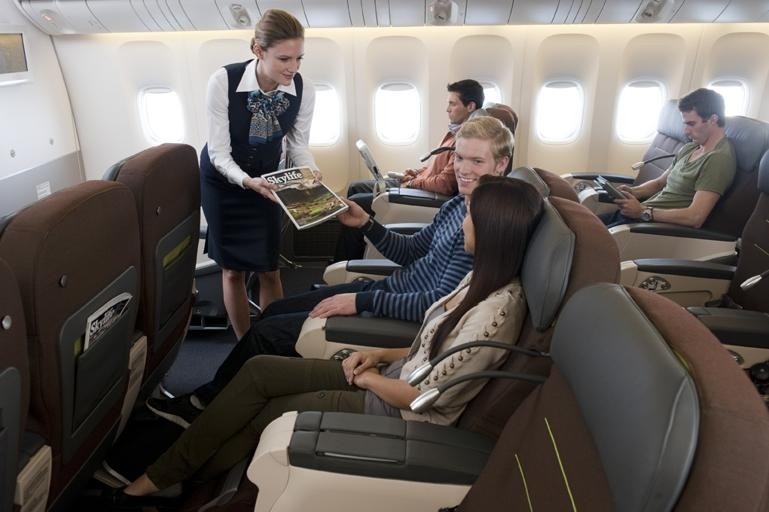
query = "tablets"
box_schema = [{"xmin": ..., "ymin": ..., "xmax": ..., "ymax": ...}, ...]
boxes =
[{"xmin": 597, "ymin": 174, "xmax": 625, "ymax": 200}]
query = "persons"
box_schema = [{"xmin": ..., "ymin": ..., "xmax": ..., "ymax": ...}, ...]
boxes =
[
  {"xmin": 100, "ymin": 116, "xmax": 516, "ymax": 486},
  {"xmin": 72, "ymin": 175, "xmax": 548, "ymax": 511},
  {"xmin": 334, "ymin": 79, "xmax": 485, "ymax": 261},
  {"xmin": 596, "ymin": 86, "xmax": 737, "ymax": 229},
  {"xmin": 196, "ymin": 9, "xmax": 325, "ymax": 343}
]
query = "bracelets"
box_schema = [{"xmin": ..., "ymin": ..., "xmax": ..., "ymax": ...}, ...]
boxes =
[{"xmin": 359, "ymin": 215, "xmax": 374, "ymax": 235}]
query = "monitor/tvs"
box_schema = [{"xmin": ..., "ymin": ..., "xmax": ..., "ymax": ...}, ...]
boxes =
[{"xmin": 0, "ymin": 24, "xmax": 36, "ymax": 87}]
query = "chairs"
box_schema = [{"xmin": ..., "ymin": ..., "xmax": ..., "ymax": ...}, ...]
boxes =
[
  {"xmin": 94, "ymin": 97, "xmax": 769, "ymax": 512},
  {"xmin": 1, "ymin": 94, "xmax": 295, "ymax": 512}
]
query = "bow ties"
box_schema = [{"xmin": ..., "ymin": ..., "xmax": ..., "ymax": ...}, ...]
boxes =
[{"xmin": 246, "ymin": 89, "xmax": 291, "ymax": 146}]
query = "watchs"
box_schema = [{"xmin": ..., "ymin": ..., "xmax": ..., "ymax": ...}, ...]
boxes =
[{"xmin": 640, "ymin": 207, "xmax": 655, "ymax": 222}]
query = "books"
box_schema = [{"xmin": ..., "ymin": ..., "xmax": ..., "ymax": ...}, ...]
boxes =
[
  {"xmin": 593, "ymin": 174, "xmax": 627, "ymax": 199},
  {"xmin": 259, "ymin": 167, "xmax": 351, "ymax": 233}
]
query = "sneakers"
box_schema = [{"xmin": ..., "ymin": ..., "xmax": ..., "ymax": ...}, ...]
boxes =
[
  {"xmin": 146, "ymin": 389, "xmax": 206, "ymax": 430},
  {"xmin": 102, "ymin": 453, "xmax": 161, "ymax": 486}
]
